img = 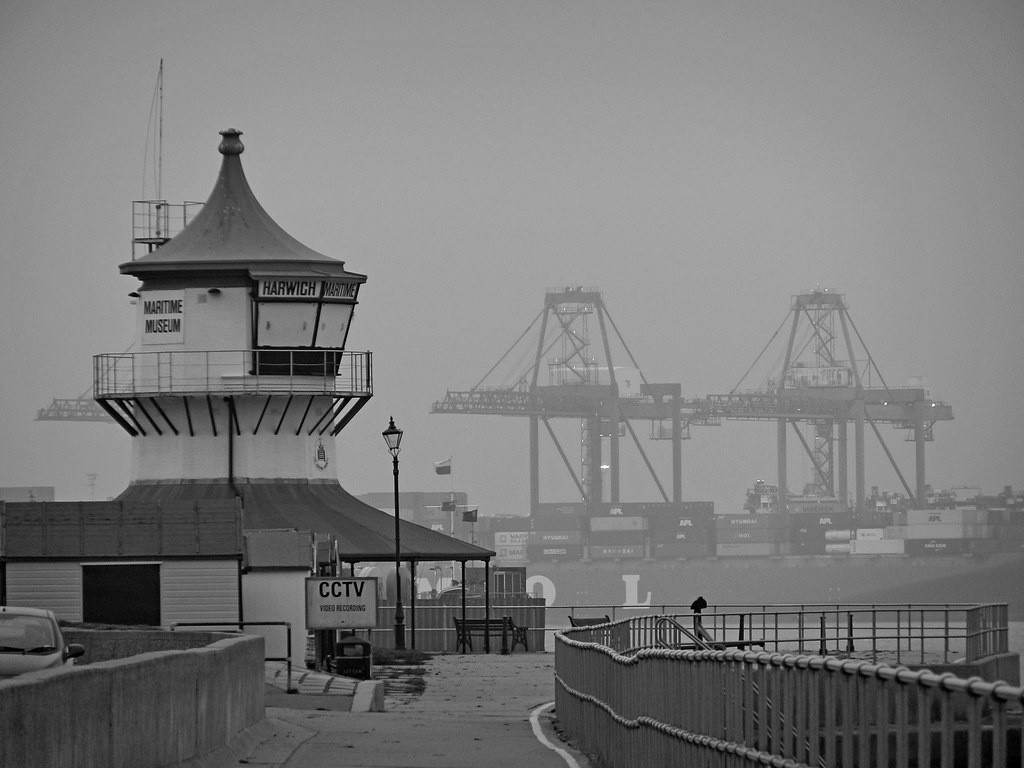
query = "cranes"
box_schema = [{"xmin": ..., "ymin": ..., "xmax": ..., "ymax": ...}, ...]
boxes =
[
  {"xmin": 677, "ymin": 291, "xmax": 958, "ymax": 558},
  {"xmin": 429, "ymin": 285, "xmax": 718, "ymax": 558}
]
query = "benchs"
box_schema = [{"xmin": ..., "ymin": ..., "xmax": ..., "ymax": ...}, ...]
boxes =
[
  {"xmin": 452, "ymin": 616, "xmax": 531, "ymax": 654},
  {"xmin": 568, "ymin": 615, "xmax": 611, "ymax": 627}
]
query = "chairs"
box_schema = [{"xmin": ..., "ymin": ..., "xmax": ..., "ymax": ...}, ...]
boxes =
[{"xmin": 16, "ymin": 624, "xmax": 50, "ymax": 650}]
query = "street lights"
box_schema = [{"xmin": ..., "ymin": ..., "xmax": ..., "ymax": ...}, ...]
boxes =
[{"xmin": 382, "ymin": 415, "xmax": 409, "ymax": 653}]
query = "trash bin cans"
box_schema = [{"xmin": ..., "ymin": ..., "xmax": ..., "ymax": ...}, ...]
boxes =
[{"xmin": 336, "ymin": 638, "xmax": 373, "ymax": 679}]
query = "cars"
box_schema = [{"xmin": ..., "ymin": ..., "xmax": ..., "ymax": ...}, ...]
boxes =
[{"xmin": 0, "ymin": 604, "xmax": 86, "ymax": 679}]
